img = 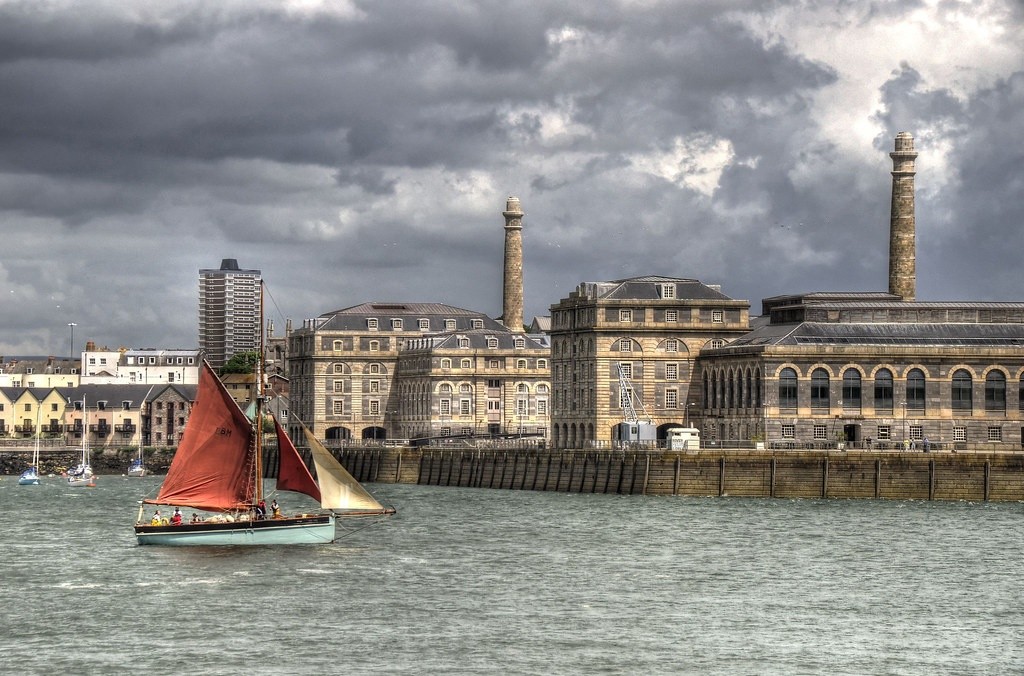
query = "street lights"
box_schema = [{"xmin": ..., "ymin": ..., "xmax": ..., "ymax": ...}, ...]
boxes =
[{"xmin": 679, "ymin": 402, "xmax": 696, "ymax": 428}]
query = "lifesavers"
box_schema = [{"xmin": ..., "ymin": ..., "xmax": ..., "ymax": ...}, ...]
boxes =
[{"xmin": 160, "ymin": 518, "xmax": 169, "ymax": 526}]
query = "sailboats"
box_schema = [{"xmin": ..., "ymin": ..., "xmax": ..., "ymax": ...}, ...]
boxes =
[
  {"xmin": 252, "ymin": 385, "xmax": 397, "ymax": 517},
  {"xmin": 18, "ymin": 403, "xmax": 42, "ymax": 485},
  {"xmin": 64, "ymin": 393, "xmax": 93, "ymax": 487},
  {"xmin": 131, "ymin": 277, "xmax": 338, "ymax": 549},
  {"xmin": 128, "ymin": 407, "xmax": 149, "ymax": 477}
]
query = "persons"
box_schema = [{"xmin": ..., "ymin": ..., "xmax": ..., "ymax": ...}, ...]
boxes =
[
  {"xmin": 151, "ymin": 506, "xmax": 202, "ymax": 525},
  {"xmin": 271, "ymin": 499, "xmax": 279, "ymax": 515},
  {"xmin": 258, "ymin": 499, "xmax": 266, "ymax": 520}
]
